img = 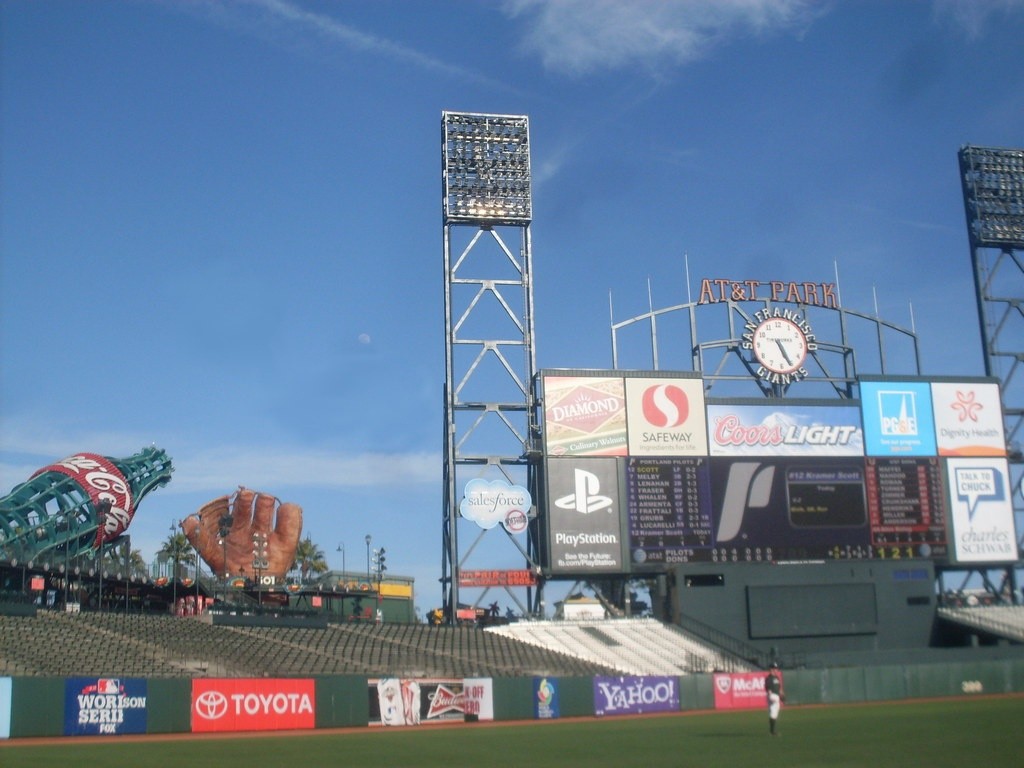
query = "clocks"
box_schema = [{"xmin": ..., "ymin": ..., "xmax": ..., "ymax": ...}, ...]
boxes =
[{"xmin": 752, "ymin": 316, "xmax": 807, "ymax": 374}]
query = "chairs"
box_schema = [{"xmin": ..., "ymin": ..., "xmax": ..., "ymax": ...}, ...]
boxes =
[
  {"xmin": 938, "ymin": 606, "xmax": 1024, "ymax": 638},
  {"xmin": 0, "ymin": 608, "xmax": 806, "ymax": 677}
]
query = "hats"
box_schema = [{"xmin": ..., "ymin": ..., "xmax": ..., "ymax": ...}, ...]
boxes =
[{"xmin": 770, "ymin": 662, "xmax": 777, "ymax": 668}]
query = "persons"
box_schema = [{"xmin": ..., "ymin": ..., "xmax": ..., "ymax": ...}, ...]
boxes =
[
  {"xmin": 344, "ymin": 584, "xmax": 352, "ymax": 595},
  {"xmin": 332, "ymin": 583, "xmax": 337, "ymax": 594},
  {"xmin": 376, "ymin": 609, "xmax": 382, "ymax": 626},
  {"xmin": 769, "ymin": 690, "xmax": 780, "ymax": 736},
  {"xmin": 317, "ymin": 582, "xmax": 324, "ymax": 593}
]
